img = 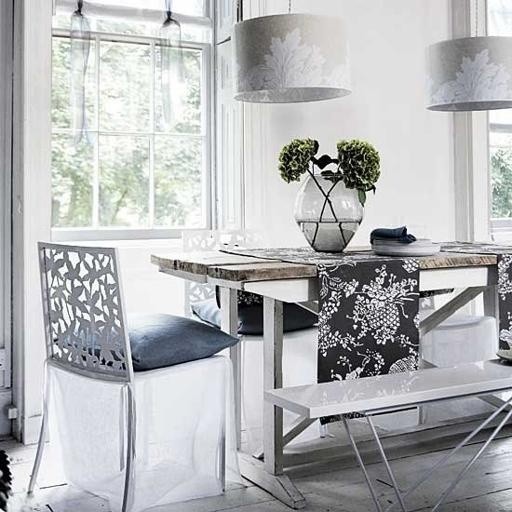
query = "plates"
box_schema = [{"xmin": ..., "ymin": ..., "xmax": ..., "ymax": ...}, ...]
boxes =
[{"xmin": 372, "ymin": 239, "xmax": 441, "ymax": 256}]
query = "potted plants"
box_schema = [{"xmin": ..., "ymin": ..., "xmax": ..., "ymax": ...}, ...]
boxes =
[{"xmin": 279, "ymin": 139, "xmax": 379, "ymax": 251}]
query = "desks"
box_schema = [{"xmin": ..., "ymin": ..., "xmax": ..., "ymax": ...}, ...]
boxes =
[{"xmin": 151, "ymin": 240, "xmax": 512, "ymax": 510}]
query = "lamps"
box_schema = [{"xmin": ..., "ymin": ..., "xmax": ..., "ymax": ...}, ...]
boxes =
[
  {"xmin": 231, "ymin": 0, "xmax": 352, "ymax": 103},
  {"xmin": 424, "ymin": 0, "xmax": 512, "ymax": 111}
]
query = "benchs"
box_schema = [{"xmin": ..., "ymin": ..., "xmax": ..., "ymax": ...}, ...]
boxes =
[{"xmin": 264, "ymin": 360, "xmax": 512, "ymax": 511}]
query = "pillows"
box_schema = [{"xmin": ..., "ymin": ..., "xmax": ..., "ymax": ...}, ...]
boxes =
[
  {"xmin": 187, "ymin": 294, "xmax": 319, "ymax": 335},
  {"xmin": 55, "ymin": 315, "xmax": 241, "ymax": 370}
]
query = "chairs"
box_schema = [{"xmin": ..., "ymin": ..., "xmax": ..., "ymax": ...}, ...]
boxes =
[{"xmin": 27, "ymin": 240, "xmax": 240, "ymax": 512}]
